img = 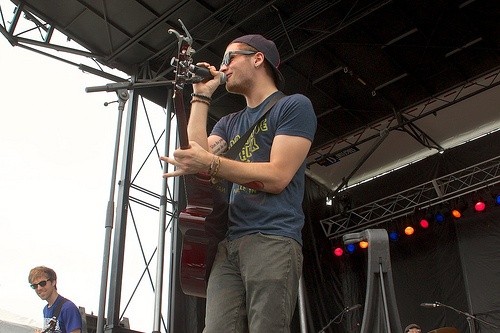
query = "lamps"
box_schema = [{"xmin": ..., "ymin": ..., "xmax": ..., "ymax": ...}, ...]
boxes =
[
  {"xmin": 449, "ymin": 202, "xmax": 469, "ymax": 219},
  {"xmin": 472, "ymin": 195, "xmax": 488, "ymax": 215},
  {"xmin": 435, "ymin": 211, "xmax": 449, "ymax": 223},
  {"xmin": 420, "ymin": 215, "xmax": 434, "ymax": 228}
]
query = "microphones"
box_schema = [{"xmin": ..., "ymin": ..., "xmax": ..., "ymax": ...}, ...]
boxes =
[
  {"xmin": 344, "ymin": 304, "xmax": 362, "ymax": 314},
  {"xmin": 419, "ymin": 303, "xmax": 443, "ymax": 308},
  {"xmin": 188, "ymin": 64, "xmax": 227, "ymax": 86}
]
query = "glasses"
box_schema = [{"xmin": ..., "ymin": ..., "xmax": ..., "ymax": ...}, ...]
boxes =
[
  {"xmin": 30, "ymin": 279, "xmax": 52, "ymax": 289},
  {"xmin": 220, "ymin": 50, "xmax": 258, "ymax": 67}
]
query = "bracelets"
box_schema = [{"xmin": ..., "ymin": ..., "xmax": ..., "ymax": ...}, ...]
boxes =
[
  {"xmin": 210, "ymin": 154, "xmax": 220, "ymax": 177},
  {"xmin": 190, "ymin": 93, "xmax": 212, "ymax": 102},
  {"xmin": 190, "ymin": 99, "xmax": 210, "ymax": 106}
]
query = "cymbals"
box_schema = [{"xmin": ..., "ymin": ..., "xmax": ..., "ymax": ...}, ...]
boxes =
[{"xmin": 429, "ymin": 326, "xmax": 460, "ymax": 333}]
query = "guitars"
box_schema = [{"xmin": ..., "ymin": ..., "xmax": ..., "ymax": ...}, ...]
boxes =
[
  {"xmin": 42, "ymin": 316, "xmax": 59, "ymax": 333},
  {"xmin": 166, "ymin": 18, "xmax": 229, "ymax": 299}
]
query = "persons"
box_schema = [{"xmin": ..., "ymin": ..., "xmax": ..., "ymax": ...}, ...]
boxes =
[
  {"xmin": 28, "ymin": 266, "xmax": 82, "ymax": 333},
  {"xmin": 159, "ymin": 35, "xmax": 318, "ymax": 333}
]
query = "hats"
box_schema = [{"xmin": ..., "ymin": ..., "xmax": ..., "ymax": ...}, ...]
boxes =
[{"xmin": 232, "ymin": 35, "xmax": 285, "ymax": 91}]
